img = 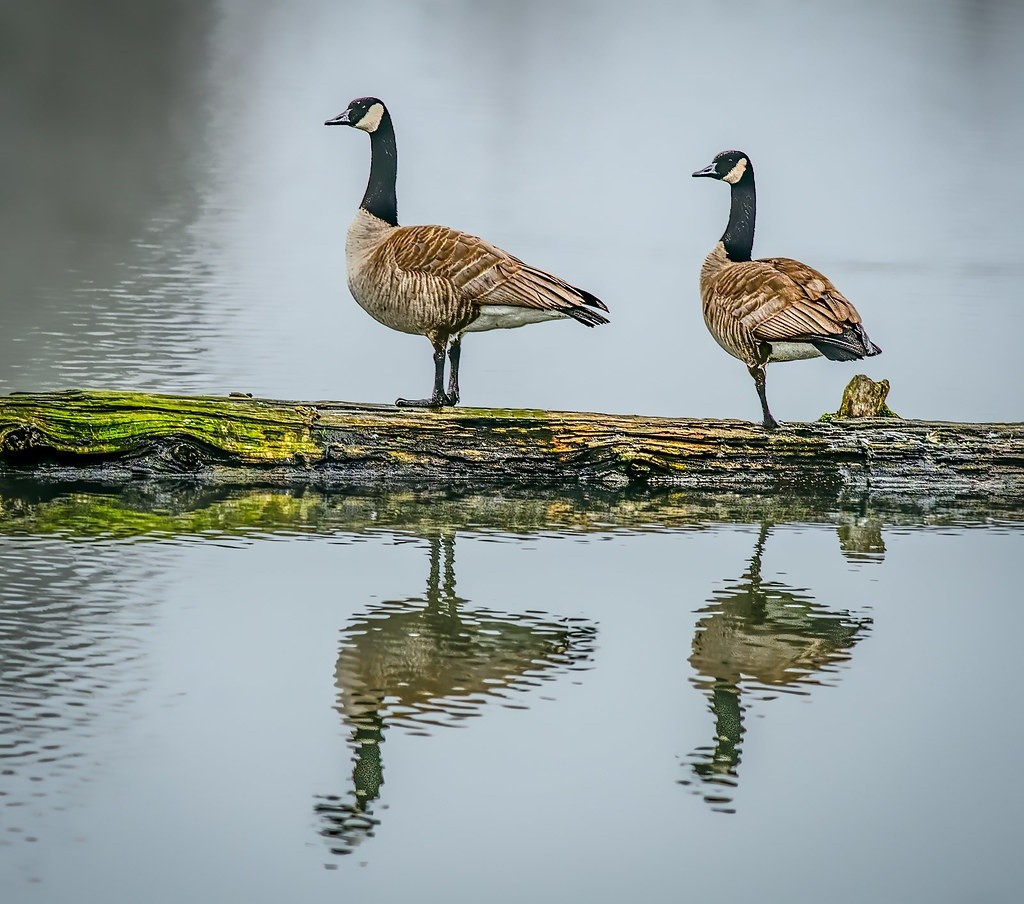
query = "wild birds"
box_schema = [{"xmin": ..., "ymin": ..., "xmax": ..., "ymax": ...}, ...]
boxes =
[
  {"xmin": 324, "ymin": 96, "xmax": 610, "ymax": 407},
  {"xmin": 683, "ymin": 517, "xmax": 876, "ymax": 818},
  {"xmin": 691, "ymin": 148, "xmax": 881, "ymax": 430},
  {"xmin": 313, "ymin": 529, "xmax": 601, "ymax": 871}
]
